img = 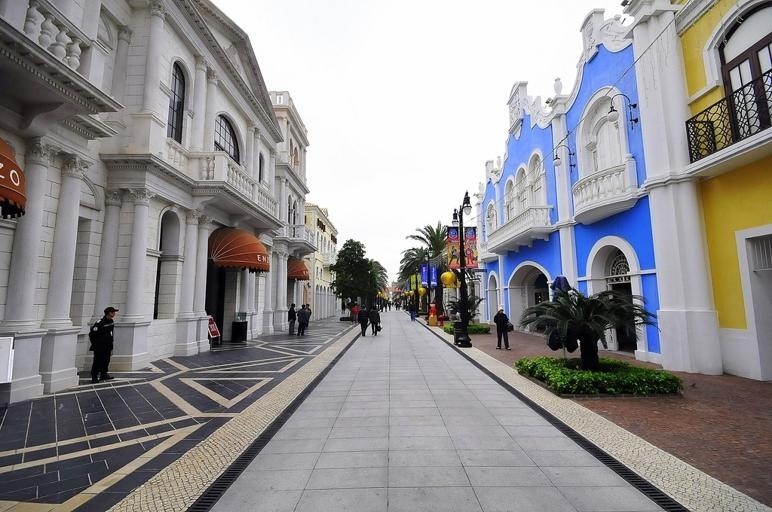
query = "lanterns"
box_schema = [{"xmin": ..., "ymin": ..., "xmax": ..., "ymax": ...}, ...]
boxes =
[
  {"xmin": 439, "ymin": 271, "xmax": 456, "ymax": 288},
  {"xmin": 377, "ymin": 288, "xmax": 426, "ymax": 300}
]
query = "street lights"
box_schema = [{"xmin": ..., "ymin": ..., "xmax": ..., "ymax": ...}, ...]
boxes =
[
  {"xmin": 367, "ymin": 270, "xmax": 372, "ymax": 313},
  {"xmin": 424, "ymin": 244, "xmax": 433, "ymax": 320},
  {"xmin": 451, "ymin": 189, "xmax": 474, "ymax": 349},
  {"xmin": 415, "ymin": 265, "xmax": 419, "ymax": 289}
]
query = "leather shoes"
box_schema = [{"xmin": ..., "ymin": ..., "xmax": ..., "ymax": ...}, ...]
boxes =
[
  {"xmin": 100, "ymin": 374, "xmax": 114, "ymax": 379},
  {"xmin": 92, "ymin": 380, "xmax": 99, "ymax": 383}
]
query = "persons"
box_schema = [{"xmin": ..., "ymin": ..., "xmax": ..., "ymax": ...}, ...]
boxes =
[
  {"xmin": 410, "ymin": 301, "xmax": 416, "ymax": 321},
  {"xmin": 369, "ymin": 305, "xmax": 380, "ymax": 336},
  {"xmin": 304, "ymin": 303, "xmax": 312, "ymax": 329},
  {"xmin": 287, "ymin": 303, "xmax": 296, "ymax": 334},
  {"xmin": 358, "ymin": 304, "xmax": 369, "ymax": 336},
  {"xmin": 379, "ymin": 299, "xmax": 401, "ymax": 312},
  {"xmin": 350, "ymin": 303, "xmax": 359, "ymax": 325},
  {"xmin": 494, "ymin": 304, "xmax": 511, "ymax": 351},
  {"xmin": 89, "ymin": 306, "xmax": 120, "ymax": 382},
  {"xmin": 297, "ymin": 305, "xmax": 308, "ymax": 336}
]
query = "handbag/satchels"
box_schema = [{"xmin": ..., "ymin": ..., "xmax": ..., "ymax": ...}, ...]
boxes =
[{"xmin": 376, "ymin": 325, "xmax": 381, "ymax": 331}]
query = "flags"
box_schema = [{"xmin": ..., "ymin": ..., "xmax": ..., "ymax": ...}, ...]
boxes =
[{"xmin": 405, "ymin": 226, "xmax": 478, "ymax": 293}]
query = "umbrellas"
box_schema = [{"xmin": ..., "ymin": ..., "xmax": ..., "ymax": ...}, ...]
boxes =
[{"xmin": 545, "ymin": 275, "xmax": 579, "ymax": 360}]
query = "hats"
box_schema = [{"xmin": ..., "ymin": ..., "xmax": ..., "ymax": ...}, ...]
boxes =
[
  {"xmin": 104, "ymin": 307, "xmax": 119, "ymax": 313},
  {"xmin": 498, "ymin": 307, "xmax": 504, "ymax": 312}
]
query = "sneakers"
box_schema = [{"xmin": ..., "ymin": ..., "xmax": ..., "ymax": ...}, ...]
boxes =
[
  {"xmin": 496, "ymin": 347, "xmax": 501, "ymax": 349},
  {"xmin": 507, "ymin": 347, "xmax": 511, "ymax": 350}
]
